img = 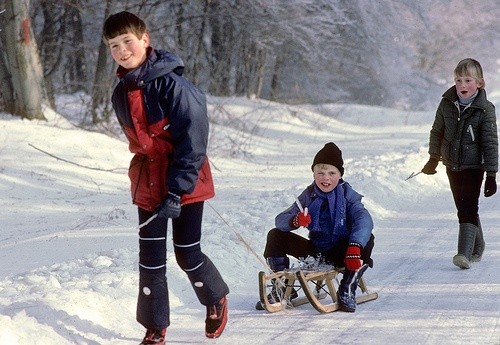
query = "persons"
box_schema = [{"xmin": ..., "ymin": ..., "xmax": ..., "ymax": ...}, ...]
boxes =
[
  {"xmin": 255, "ymin": 142, "xmax": 375, "ymax": 311},
  {"xmin": 421, "ymin": 58, "xmax": 498, "ymax": 269},
  {"xmin": 103, "ymin": 10, "xmax": 230, "ymax": 345}
]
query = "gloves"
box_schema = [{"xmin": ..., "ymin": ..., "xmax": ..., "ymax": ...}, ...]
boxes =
[
  {"xmin": 484, "ymin": 176, "xmax": 497, "ymax": 197},
  {"xmin": 343, "ymin": 242, "xmax": 361, "ymax": 271},
  {"xmin": 422, "ymin": 158, "xmax": 438, "ymax": 175},
  {"xmin": 293, "ymin": 211, "xmax": 311, "ymax": 227},
  {"xmin": 153, "ymin": 191, "xmax": 181, "ymax": 218}
]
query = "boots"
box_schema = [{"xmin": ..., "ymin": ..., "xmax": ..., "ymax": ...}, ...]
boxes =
[
  {"xmin": 453, "ymin": 223, "xmax": 477, "ymax": 269},
  {"xmin": 337, "ymin": 260, "xmax": 369, "ymax": 312},
  {"xmin": 472, "ymin": 215, "xmax": 486, "ymax": 262},
  {"xmin": 256, "ymin": 256, "xmax": 298, "ymax": 310}
]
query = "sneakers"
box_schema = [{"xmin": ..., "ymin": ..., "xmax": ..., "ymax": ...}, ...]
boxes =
[
  {"xmin": 139, "ymin": 328, "xmax": 166, "ymax": 345},
  {"xmin": 205, "ymin": 295, "xmax": 228, "ymax": 339}
]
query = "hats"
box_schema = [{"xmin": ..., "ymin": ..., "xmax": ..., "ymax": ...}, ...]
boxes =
[{"xmin": 311, "ymin": 142, "xmax": 344, "ymax": 177}]
